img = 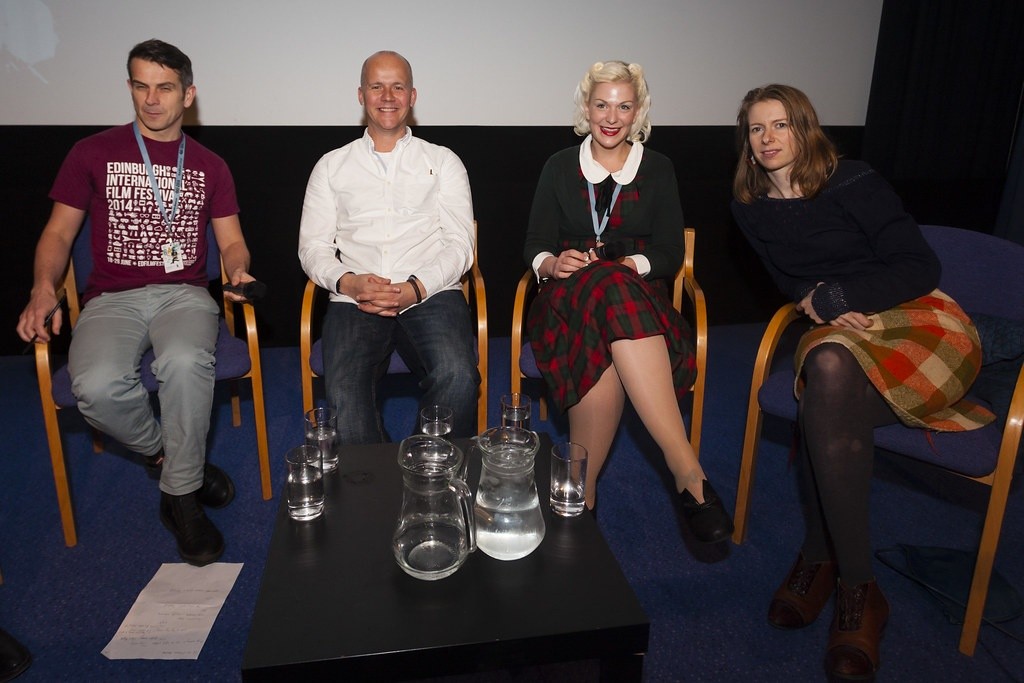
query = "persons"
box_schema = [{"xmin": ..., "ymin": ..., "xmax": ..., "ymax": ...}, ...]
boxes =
[
  {"xmin": 299, "ymin": 51, "xmax": 482, "ymax": 447},
  {"xmin": 16, "ymin": 38, "xmax": 257, "ymax": 568},
  {"xmin": 523, "ymin": 61, "xmax": 736, "ymax": 544},
  {"xmin": 736, "ymin": 85, "xmax": 983, "ymax": 683}
]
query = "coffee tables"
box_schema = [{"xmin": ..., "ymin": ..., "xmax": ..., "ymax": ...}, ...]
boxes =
[{"xmin": 242, "ymin": 432, "xmax": 651, "ymax": 683}]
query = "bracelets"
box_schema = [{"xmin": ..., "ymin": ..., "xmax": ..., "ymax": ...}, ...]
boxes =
[
  {"xmin": 336, "ymin": 271, "xmax": 355, "ymax": 294},
  {"xmin": 406, "ymin": 278, "xmax": 422, "ymax": 304}
]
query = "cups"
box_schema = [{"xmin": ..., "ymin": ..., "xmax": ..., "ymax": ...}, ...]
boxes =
[
  {"xmin": 500, "ymin": 392, "xmax": 532, "ymax": 431},
  {"xmin": 285, "ymin": 444, "xmax": 326, "ymax": 523},
  {"xmin": 304, "ymin": 407, "xmax": 340, "ymax": 474},
  {"xmin": 419, "ymin": 405, "xmax": 454, "ymax": 443},
  {"xmin": 549, "ymin": 442, "xmax": 589, "ymax": 518}
]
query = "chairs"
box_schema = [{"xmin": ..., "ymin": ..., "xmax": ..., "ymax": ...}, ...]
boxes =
[
  {"xmin": 34, "ymin": 208, "xmax": 273, "ymax": 549},
  {"xmin": 509, "ymin": 196, "xmax": 707, "ymax": 461},
  {"xmin": 732, "ymin": 224, "xmax": 1024, "ymax": 657},
  {"xmin": 301, "ymin": 217, "xmax": 489, "ymax": 437}
]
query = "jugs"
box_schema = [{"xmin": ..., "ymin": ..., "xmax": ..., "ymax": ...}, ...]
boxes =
[
  {"xmin": 392, "ymin": 434, "xmax": 477, "ymax": 582},
  {"xmin": 460, "ymin": 426, "xmax": 546, "ymax": 561}
]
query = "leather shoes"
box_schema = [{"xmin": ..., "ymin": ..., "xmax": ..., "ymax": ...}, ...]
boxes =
[
  {"xmin": 589, "ymin": 490, "xmax": 597, "ymax": 519},
  {"xmin": 679, "ymin": 478, "xmax": 733, "ymax": 545},
  {"xmin": 147, "ymin": 453, "xmax": 234, "ymax": 509},
  {"xmin": 825, "ymin": 577, "xmax": 892, "ymax": 683},
  {"xmin": 159, "ymin": 492, "xmax": 225, "ymax": 565},
  {"xmin": 767, "ymin": 549, "xmax": 838, "ymax": 630}
]
